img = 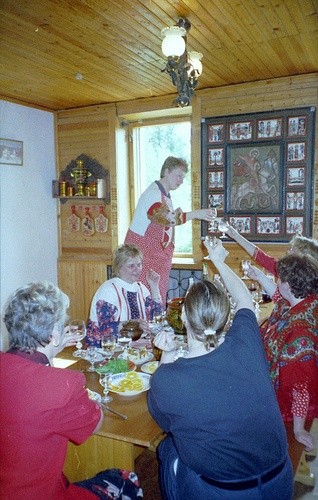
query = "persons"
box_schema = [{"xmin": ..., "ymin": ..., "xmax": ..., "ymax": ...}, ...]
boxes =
[
  {"xmin": 242, "ymin": 234, "xmax": 318, "ymax": 330},
  {"xmin": 146, "ymin": 238, "xmax": 294, "ymax": 500},
  {"xmin": 0, "ymin": 281, "xmax": 144, "ymax": 500},
  {"xmin": 208, "ymin": 218, "xmax": 318, "ymax": 452},
  {"xmin": 84, "ymin": 243, "xmax": 163, "ymax": 350},
  {"xmin": 124, "ymin": 156, "xmax": 215, "ymax": 312}
]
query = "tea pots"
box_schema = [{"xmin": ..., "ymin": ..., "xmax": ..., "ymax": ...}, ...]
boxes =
[
  {"xmin": 166, "ymin": 297, "xmax": 187, "ymax": 334},
  {"xmin": 119, "ymin": 321, "xmax": 143, "ymax": 341}
]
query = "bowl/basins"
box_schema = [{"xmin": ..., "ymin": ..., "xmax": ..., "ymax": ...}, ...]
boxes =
[{"xmin": 99, "ymin": 371, "xmax": 153, "ymax": 397}]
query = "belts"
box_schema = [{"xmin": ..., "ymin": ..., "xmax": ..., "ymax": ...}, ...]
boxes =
[{"xmin": 197, "ymin": 454, "xmax": 288, "ymax": 491}]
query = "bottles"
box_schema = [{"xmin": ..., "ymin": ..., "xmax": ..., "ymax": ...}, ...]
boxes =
[{"xmin": 183, "ymin": 263, "xmax": 228, "ymax": 298}]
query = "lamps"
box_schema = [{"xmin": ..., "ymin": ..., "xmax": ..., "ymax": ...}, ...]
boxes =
[{"xmin": 159, "ymin": 16, "xmax": 203, "ymax": 109}]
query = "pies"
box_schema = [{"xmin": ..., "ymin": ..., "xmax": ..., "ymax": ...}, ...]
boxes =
[{"xmin": 113, "ymin": 372, "xmax": 143, "ymax": 392}]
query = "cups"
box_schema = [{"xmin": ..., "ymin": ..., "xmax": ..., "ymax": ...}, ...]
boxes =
[{"xmin": 102, "ymin": 335, "xmax": 116, "ymax": 363}]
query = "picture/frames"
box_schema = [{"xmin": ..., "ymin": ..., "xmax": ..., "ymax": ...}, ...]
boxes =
[
  {"xmin": 200, "ymin": 106, "xmax": 316, "ymax": 245},
  {"xmin": 0, "ymin": 138, "xmax": 23, "ymax": 166}
]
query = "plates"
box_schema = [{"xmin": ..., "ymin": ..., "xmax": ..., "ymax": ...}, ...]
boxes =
[
  {"xmin": 71, "ymin": 348, "xmax": 117, "ymax": 361},
  {"xmin": 141, "ymin": 361, "xmax": 159, "ymax": 375},
  {"xmin": 96, "ymin": 360, "xmax": 137, "ymax": 376},
  {"xmin": 118, "ymin": 351, "xmax": 154, "ymax": 366}
]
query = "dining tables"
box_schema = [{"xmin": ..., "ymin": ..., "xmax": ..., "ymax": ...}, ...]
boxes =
[{"xmin": 49, "ymin": 326, "xmax": 166, "ymax": 482}]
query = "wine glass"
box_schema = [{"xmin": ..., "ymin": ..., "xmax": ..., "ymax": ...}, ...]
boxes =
[
  {"xmin": 94, "ymin": 350, "xmax": 116, "ymax": 404},
  {"xmin": 68, "ymin": 319, "xmax": 88, "ymax": 358},
  {"xmin": 116, "ymin": 329, "xmax": 133, "ymax": 358},
  {"xmin": 248, "ymin": 281, "xmax": 258, "ymax": 303},
  {"xmin": 203, "ymin": 235, "xmax": 218, "ymax": 260},
  {"xmin": 241, "ymin": 259, "xmax": 251, "ymax": 280},
  {"xmin": 153, "ymin": 309, "xmax": 164, "ymax": 335},
  {"xmin": 207, "ymin": 207, "xmax": 219, "ymax": 230},
  {"xmin": 86, "ymin": 346, "xmax": 98, "ymax": 373},
  {"xmin": 217, "ymin": 216, "xmax": 229, "ymax": 239}
]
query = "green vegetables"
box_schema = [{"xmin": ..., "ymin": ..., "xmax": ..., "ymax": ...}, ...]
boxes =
[{"xmin": 96, "ymin": 360, "xmax": 129, "ymax": 373}]
